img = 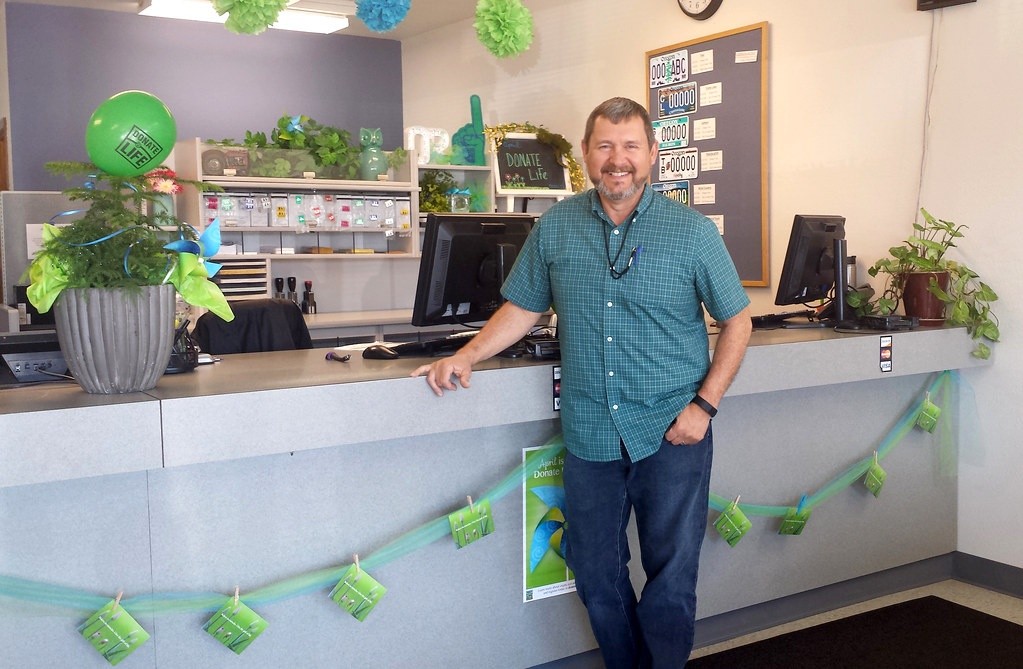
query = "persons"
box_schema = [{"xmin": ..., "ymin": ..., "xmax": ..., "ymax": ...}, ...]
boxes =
[{"xmin": 410, "ymin": 97, "xmax": 754, "ymax": 669}]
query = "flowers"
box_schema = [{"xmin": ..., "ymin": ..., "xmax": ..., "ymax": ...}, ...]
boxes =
[{"xmin": 142, "ymin": 164, "xmax": 184, "ymax": 194}]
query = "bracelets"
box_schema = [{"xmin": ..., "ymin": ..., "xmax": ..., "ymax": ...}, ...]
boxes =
[{"xmin": 690, "ymin": 394, "xmax": 718, "ymax": 418}]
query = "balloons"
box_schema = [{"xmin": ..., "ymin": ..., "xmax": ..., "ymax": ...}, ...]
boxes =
[{"xmin": 86, "ymin": 90, "xmax": 177, "ymax": 179}]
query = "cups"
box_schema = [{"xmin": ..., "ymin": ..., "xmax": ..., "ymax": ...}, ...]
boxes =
[{"xmin": 847, "ymin": 255, "xmax": 857, "ymax": 291}]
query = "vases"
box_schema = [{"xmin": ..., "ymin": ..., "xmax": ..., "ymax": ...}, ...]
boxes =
[
  {"xmin": 357, "ymin": 127, "xmax": 389, "ymax": 181},
  {"xmin": 151, "ymin": 193, "xmax": 177, "ymax": 227}
]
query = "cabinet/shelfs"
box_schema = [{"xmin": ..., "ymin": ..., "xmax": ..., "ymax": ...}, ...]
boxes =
[{"xmin": 172, "ymin": 139, "xmax": 422, "ymax": 257}]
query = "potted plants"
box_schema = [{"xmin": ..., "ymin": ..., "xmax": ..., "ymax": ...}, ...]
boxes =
[
  {"xmin": 417, "ymin": 169, "xmax": 462, "ymax": 251},
  {"xmin": 36, "ymin": 159, "xmax": 226, "ymax": 396},
  {"xmin": 845, "ymin": 206, "xmax": 999, "ymax": 363}
]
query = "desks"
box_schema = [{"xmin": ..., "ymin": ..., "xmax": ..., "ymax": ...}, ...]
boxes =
[{"xmin": 296, "ymin": 307, "xmax": 415, "ymax": 342}]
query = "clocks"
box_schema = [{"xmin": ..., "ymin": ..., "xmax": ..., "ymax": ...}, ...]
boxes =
[{"xmin": 676, "ymin": 0, "xmax": 723, "ymax": 20}]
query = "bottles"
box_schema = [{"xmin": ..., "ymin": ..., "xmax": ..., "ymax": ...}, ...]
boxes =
[{"xmin": 451, "ymin": 193, "xmax": 469, "ymax": 214}]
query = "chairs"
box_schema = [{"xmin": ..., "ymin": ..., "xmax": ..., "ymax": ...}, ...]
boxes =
[{"xmin": 190, "ymin": 298, "xmax": 314, "ymax": 355}]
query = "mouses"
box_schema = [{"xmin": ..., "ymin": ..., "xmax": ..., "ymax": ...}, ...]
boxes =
[{"xmin": 362, "ymin": 345, "xmax": 399, "ymax": 359}]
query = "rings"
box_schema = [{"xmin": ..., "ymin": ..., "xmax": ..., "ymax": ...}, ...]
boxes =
[{"xmin": 682, "ymin": 440, "xmax": 686, "ymax": 445}]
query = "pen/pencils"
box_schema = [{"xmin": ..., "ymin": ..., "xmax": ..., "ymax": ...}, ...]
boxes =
[{"xmin": 173, "ymin": 320, "xmax": 191, "ymax": 346}]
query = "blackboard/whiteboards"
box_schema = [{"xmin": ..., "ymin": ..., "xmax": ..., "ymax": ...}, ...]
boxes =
[
  {"xmin": 644, "ymin": 21, "xmax": 770, "ymax": 288},
  {"xmin": 490, "ymin": 132, "xmax": 574, "ymax": 198}
]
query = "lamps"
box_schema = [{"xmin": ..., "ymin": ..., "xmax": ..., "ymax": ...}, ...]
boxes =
[{"xmin": 137, "ymin": 0, "xmax": 350, "ymax": 35}]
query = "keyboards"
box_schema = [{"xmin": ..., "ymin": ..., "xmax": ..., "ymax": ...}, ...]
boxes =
[
  {"xmin": 751, "ymin": 310, "xmax": 815, "ymax": 326},
  {"xmin": 390, "ymin": 331, "xmax": 480, "ymax": 355}
]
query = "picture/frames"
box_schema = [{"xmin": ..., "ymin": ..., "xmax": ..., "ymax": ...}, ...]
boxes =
[
  {"xmin": 488, "ymin": 132, "xmax": 573, "ymax": 199},
  {"xmin": 645, "ymin": 21, "xmax": 770, "ymax": 289}
]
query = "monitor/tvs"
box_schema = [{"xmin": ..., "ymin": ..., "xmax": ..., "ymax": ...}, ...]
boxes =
[
  {"xmin": 412, "ymin": 212, "xmax": 534, "ymax": 358},
  {"xmin": 774, "ymin": 215, "xmax": 859, "ymax": 329}
]
